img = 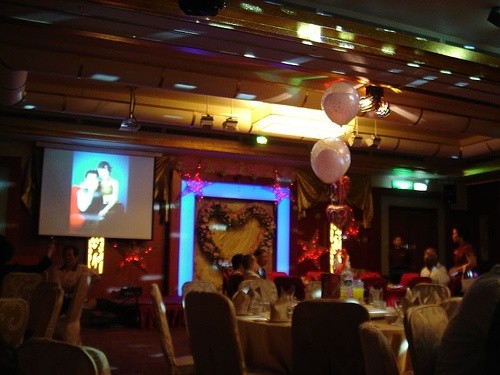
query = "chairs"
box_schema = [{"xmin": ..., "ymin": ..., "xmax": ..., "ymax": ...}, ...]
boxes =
[
  {"xmin": 151, "ymin": 272, "xmax": 468, "ymax": 375},
  {"xmin": 0, "ymin": 264, "xmax": 109, "ymax": 374}
]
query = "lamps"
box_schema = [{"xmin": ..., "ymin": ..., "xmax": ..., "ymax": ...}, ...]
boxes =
[
  {"xmin": 200, "ymin": 115, "xmax": 213, "ymax": 128},
  {"xmin": 224, "ymin": 119, "xmax": 238, "ymax": 131},
  {"xmin": 359, "ymin": 88, "xmax": 390, "ymax": 118}
]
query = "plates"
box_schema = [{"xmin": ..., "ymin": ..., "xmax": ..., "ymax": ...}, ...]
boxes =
[{"xmin": 269, "ymin": 320, "xmax": 290, "ymax": 322}]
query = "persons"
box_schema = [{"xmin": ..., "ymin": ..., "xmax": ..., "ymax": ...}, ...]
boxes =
[
  {"xmin": 433, "ymin": 265, "xmax": 500, "ymax": 375},
  {"xmin": 79, "ymin": 160, "xmax": 123, "ymax": 216},
  {"xmin": 227, "ymin": 249, "xmax": 273, "ymax": 300},
  {"xmin": 0, "ymin": 240, "xmax": 93, "ymax": 317},
  {"xmin": 387, "ymin": 226, "xmax": 479, "ymax": 283}
]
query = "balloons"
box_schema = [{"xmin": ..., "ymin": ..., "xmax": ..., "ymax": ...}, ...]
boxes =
[
  {"xmin": 321, "ymin": 82, "xmax": 361, "ymax": 126},
  {"xmin": 310, "ymin": 138, "xmax": 351, "ymax": 184}
]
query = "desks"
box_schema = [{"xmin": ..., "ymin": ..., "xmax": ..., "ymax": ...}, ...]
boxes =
[{"xmin": 239, "ymin": 307, "xmax": 409, "ymax": 375}]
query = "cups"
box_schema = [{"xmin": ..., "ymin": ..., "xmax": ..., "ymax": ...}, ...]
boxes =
[
  {"xmin": 372, "ymin": 290, "xmax": 383, "ymax": 304},
  {"xmin": 353, "ymin": 282, "xmax": 364, "ymax": 302},
  {"xmin": 282, "ymin": 284, "xmax": 295, "ymax": 302}
]
